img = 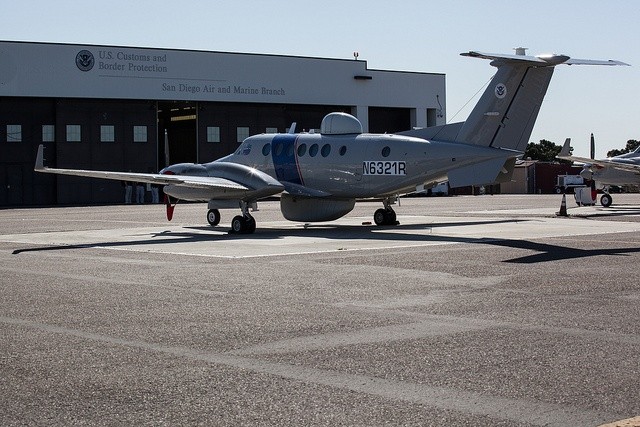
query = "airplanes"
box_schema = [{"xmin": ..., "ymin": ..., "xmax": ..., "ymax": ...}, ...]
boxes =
[
  {"xmin": 32, "ymin": 46, "xmax": 633, "ymax": 234},
  {"xmin": 554, "ymin": 137, "xmax": 640, "ymax": 207}
]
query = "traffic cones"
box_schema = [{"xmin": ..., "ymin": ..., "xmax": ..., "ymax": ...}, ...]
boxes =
[{"xmin": 555, "ymin": 193, "xmax": 570, "ymax": 217}]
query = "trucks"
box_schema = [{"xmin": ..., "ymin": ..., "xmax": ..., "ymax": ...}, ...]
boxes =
[{"xmin": 555, "ymin": 171, "xmax": 587, "ymax": 193}]
